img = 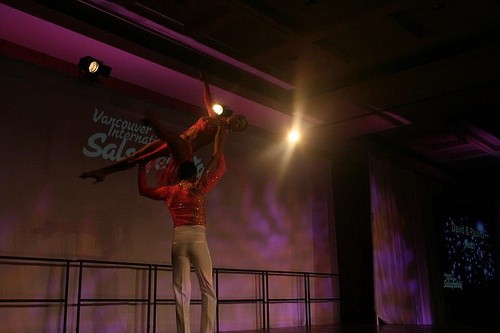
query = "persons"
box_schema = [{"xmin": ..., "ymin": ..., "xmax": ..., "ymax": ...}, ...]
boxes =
[
  {"xmin": 136, "ymin": 114, "xmax": 228, "ymax": 333},
  {"xmin": 79, "ymin": 78, "xmax": 249, "ymax": 191}
]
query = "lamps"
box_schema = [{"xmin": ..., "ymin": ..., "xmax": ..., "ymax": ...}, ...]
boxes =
[
  {"xmin": 212, "ymin": 103, "xmax": 233, "ymax": 120},
  {"xmin": 78, "ymin": 55, "xmax": 113, "ymax": 85}
]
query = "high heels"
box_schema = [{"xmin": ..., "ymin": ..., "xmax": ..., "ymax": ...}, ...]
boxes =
[{"xmin": 79, "ymin": 170, "xmax": 105, "ymax": 185}]
